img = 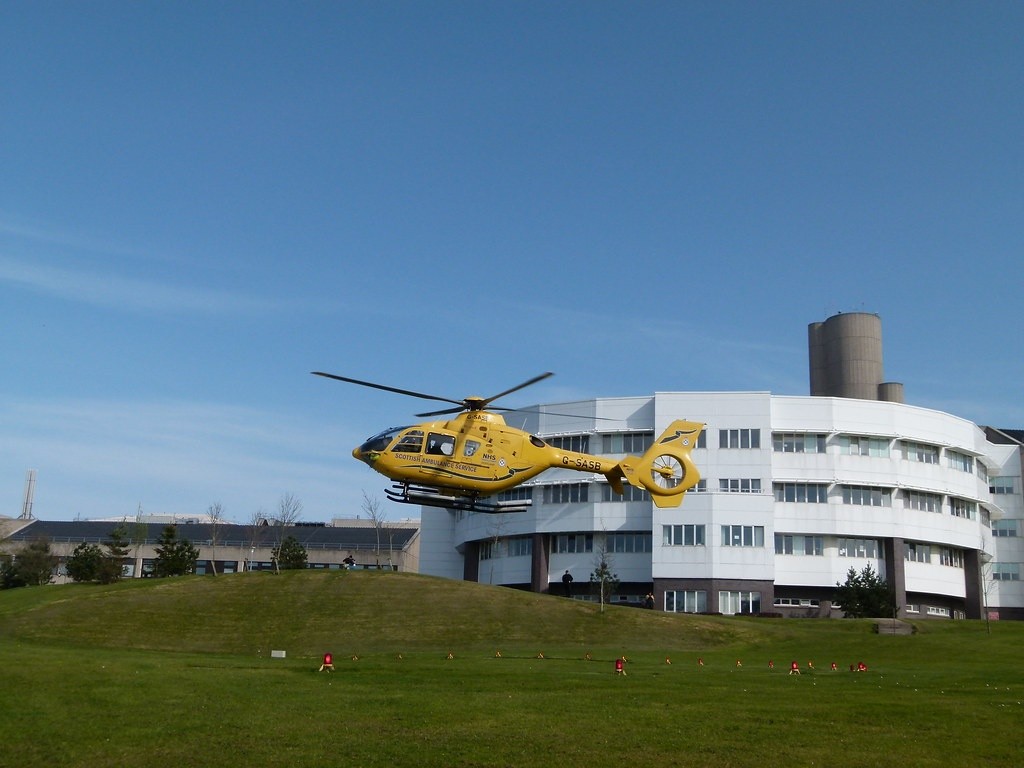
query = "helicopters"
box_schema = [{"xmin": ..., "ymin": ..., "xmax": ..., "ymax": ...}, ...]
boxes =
[{"xmin": 305, "ymin": 366, "xmax": 708, "ymax": 520}]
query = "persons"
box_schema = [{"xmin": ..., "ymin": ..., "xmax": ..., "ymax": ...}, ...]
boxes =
[
  {"xmin": 646, "ymin": 592, "xmax": 655, "ymax": 608},
  {"xmin": 562, "ymin": 570, "xmax": 573, "ymax": 598}
]
